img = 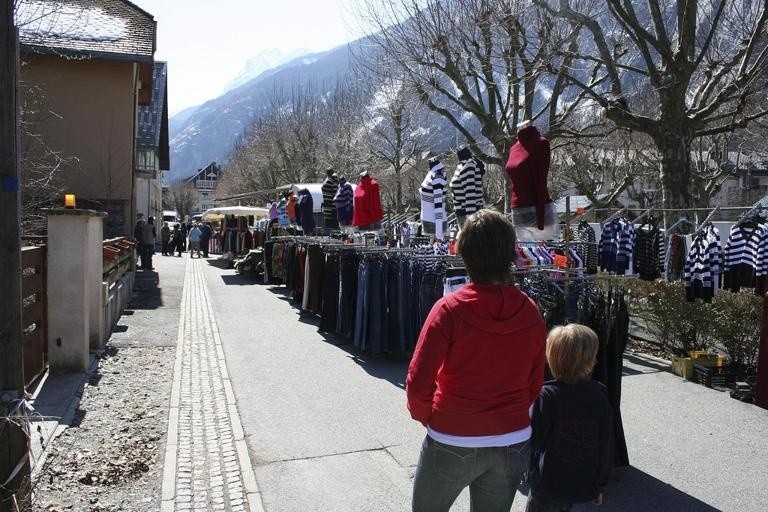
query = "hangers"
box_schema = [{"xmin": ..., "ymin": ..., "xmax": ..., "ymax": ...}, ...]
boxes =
[{"xmin": 578, "ymin": 206, "xmax": 768, "ymax": 237}]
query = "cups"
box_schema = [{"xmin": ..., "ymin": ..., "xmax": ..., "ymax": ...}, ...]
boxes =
[{"xmin": 65, "ymin": 194, "xmax": 75, "ymax": 209}]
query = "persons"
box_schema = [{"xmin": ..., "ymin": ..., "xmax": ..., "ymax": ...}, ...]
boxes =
[
  {"xmin": 265, "ymin": 165, "xmax": 384, "ymax": 235},
  {"xmin": 526, "ymin": 321, "xmax": 623, "ymax": 510},
  {"xmin": 130, "ymin": 188, "xmax": 214, "ymax": 271},
  {"xmin": 404, "ymin": 205, "xmax": 543, "ymax": 511},
  {"xmin": 448, "ymin": 143, "xmax": 486, "ymax": 231},
  {"xmin": 418, "ymin": 155, "xmax": 448, "ymax": 242},
  {"xmin": 503, "ymin": 119, "xmax": 562, "ymax": 242}
]
